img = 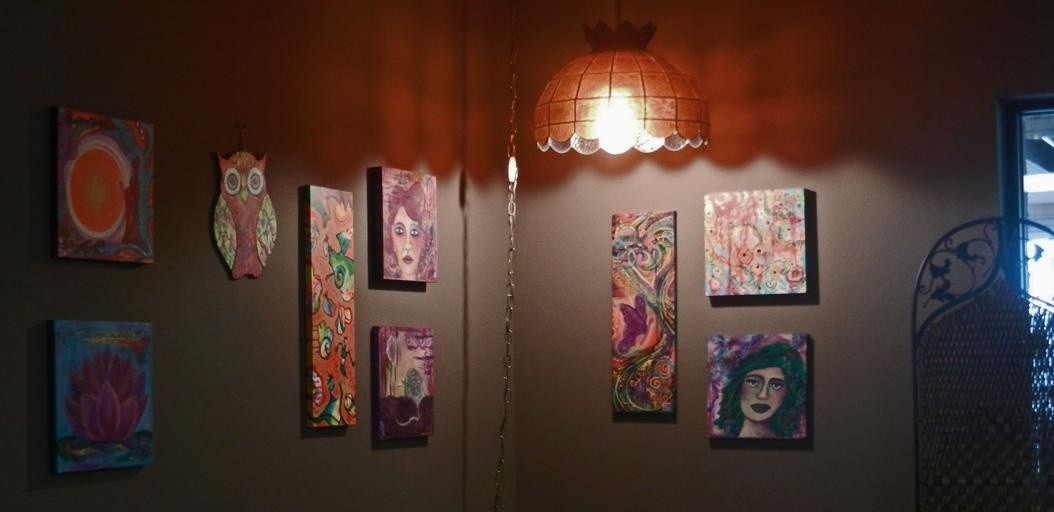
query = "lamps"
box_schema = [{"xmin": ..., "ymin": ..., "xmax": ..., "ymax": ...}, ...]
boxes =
[{"xmin": 531, "ymin": 1, "xmax": 712, "ymax": 157}]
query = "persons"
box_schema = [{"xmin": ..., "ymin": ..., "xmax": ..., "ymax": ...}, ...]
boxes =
[
  {"xmin": 717, "ymin": 341, "xmax": 804, "ymax": 438},
  {"xmin": 384, "ymin": 181, "xmax": 431, "ymax": 280}
]
